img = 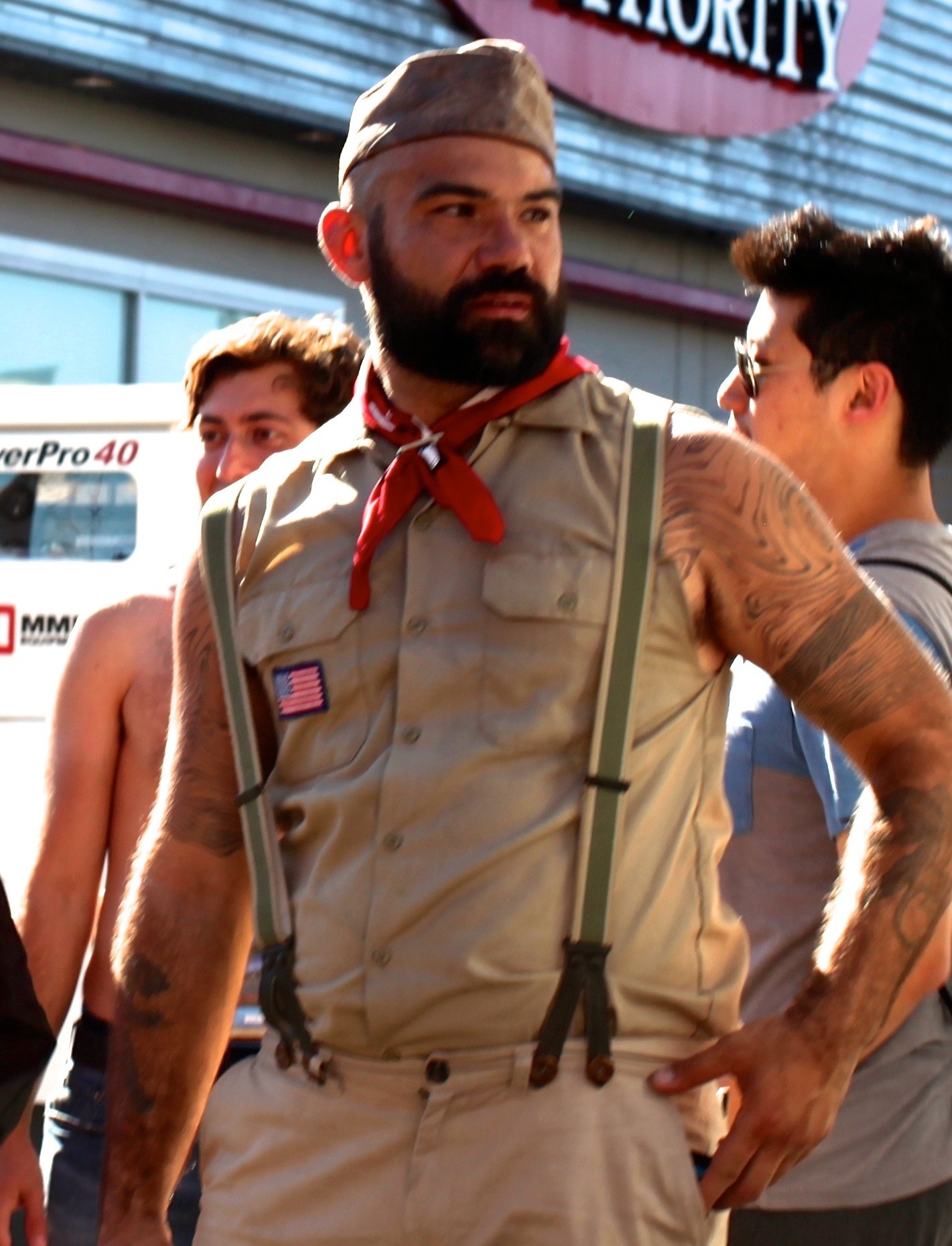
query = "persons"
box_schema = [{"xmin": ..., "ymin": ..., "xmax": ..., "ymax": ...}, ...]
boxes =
[
  {"xmin": 105, "ymin": 39, "xmax": 951, "ymax": 1246},
  {"xmin": 715, "ymin": 205, "xmax": 952, "ymax": 1246},
  {"xmin": 0, "ymin": 308, "xmax": 367, "ymax": 1246}
]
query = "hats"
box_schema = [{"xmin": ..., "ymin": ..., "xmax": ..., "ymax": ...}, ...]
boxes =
[{"xmin": 340, "ymin": 39, "xmax": 558, "ymax": 177}]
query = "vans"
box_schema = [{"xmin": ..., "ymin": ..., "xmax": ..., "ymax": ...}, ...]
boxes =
[{"xmin": 0, "ymin": 381, "xmax": 202, "ymax": 927}]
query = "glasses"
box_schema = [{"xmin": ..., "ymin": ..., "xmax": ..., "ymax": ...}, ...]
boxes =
[{"xmin": 729, "ymin": 335, "xmax": 833, "ymax": 398}]
